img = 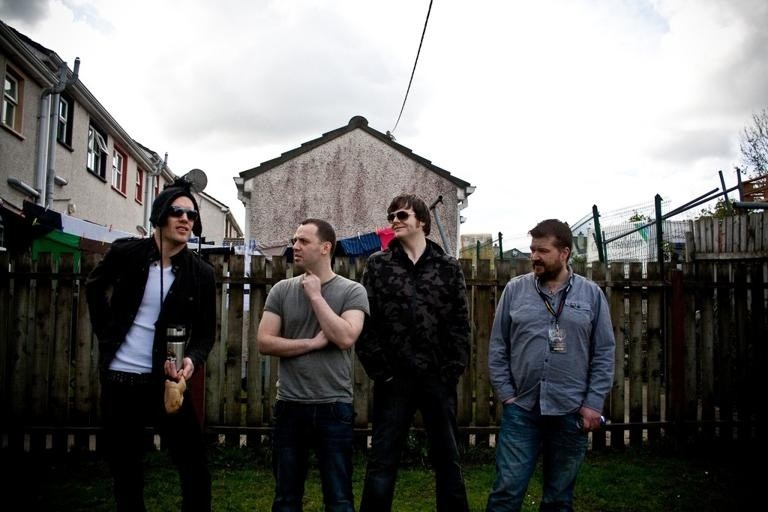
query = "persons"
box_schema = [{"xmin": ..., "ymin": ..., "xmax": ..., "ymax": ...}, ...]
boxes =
[
  {"xmin": 256, "ymin": 219, "xmax": 372, "ymax": 512},
  {"xmin": 82, "ymin": 187, "xmax": 218, "ymax": 511},
  {"xmin": 484, "ymin": 218, "xmax": 617, "ymax": 511},
  {"xmin": 354, "ymin": 193, "xmax": 474, "ymax": 512}
]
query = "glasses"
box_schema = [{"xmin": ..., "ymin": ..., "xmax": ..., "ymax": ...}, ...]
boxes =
[
  {"xmin": 386, "ymin": 211, "xmax": 417, "ymax": 223},
  {"xmin": 168, "ymin": 205, "xmax": 199, "ymax": 223}
]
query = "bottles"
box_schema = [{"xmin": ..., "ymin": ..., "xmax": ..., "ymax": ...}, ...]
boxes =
[{"xmin": 167, "ymin": 324, "xmax": 186, "ymax": 374}]
